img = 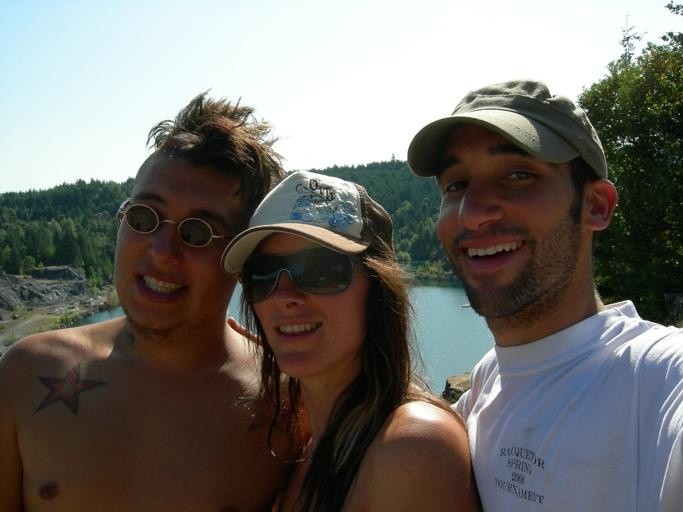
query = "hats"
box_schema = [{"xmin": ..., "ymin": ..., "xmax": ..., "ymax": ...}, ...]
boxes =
[
  {"xmin": 406, "ymin": 80, "xmax": 608, "ymax": 182},
  {"xmin": 218, "ymin": 170, "xmax": 395, "ymax": 286}
]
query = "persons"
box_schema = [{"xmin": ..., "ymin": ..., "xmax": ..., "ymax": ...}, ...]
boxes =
[
  {"xmin": 218, "ymin": 167, "xmax": 481, "ymax": 512},
  {"xmin": 227, "ymin": 77, "xmax": 683, "ymax": 512},
  {"xmin": 1, "ymin": 91, "xmax": 312, "ymax": 511}
]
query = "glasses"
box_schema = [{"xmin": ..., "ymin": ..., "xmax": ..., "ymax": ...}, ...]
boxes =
[
  {"xmin": 115, "ymin": 198, "xmax": 230, "ymax": 248},
  {"xmin": 242, "ymin": 246, "xmax": 353, "ymax": 305}
]
